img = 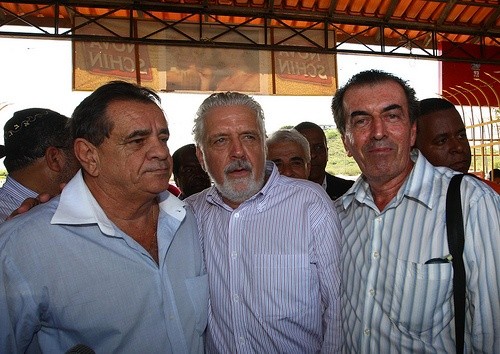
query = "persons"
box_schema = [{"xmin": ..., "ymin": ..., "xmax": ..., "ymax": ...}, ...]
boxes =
[
  {"xmin": 0, "ymin": 107, "xmax": 82, "ymax": 227},
  {"xmin": 331, "ymin": 69, "xmax": 500, "ymax": 354},
  {"xmin": 0, "ymin": 80, "xmax": 210, "ymax": 354},
  {"xmin": 413, "ymin": 98, "xmax": 500, "ymax": 195},
  {"xmin": 172, "ymin": 143, "xmax": 211, "ymax": 201},
  {"xmin": 182, "ymin": 91, "xmax": 342, "ymax": 354},
  {"xmin": 266, "ymin": 121, "xmax": 355, "ymax": 202}
]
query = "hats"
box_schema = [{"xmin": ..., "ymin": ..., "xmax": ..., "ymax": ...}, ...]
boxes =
[{"xmin": 0, "ymin": 108, "xmax": 73, "ymax": 160}]
